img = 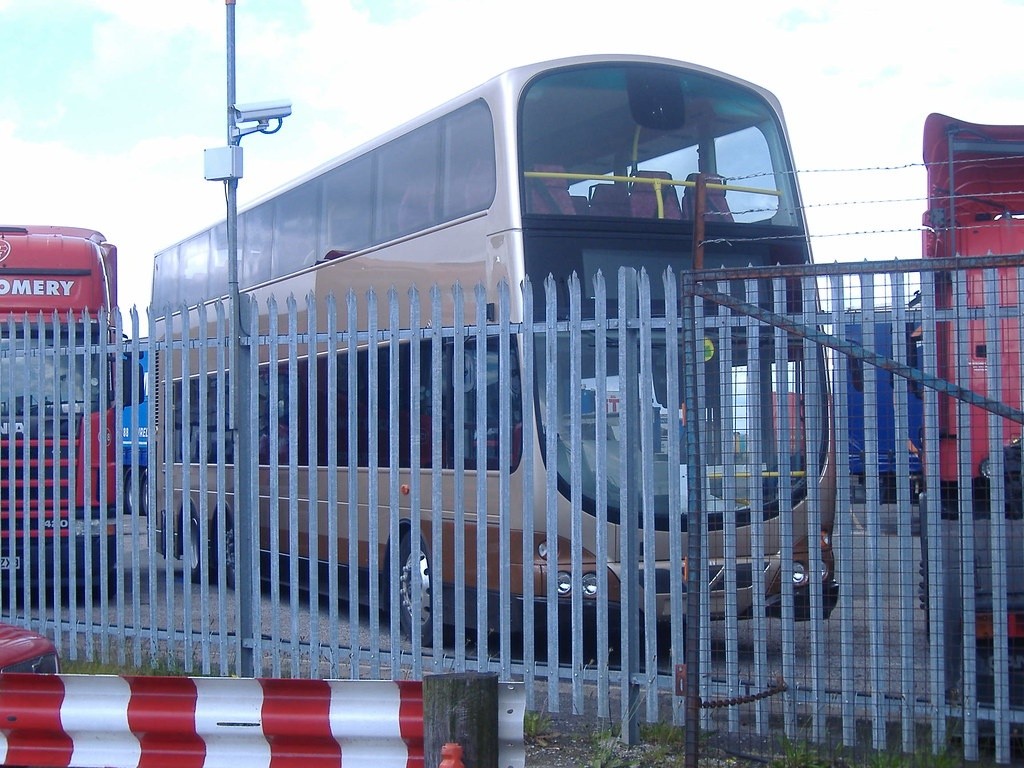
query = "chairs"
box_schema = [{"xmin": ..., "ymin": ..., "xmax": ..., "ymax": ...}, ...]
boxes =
[{"xmin": 182, "ymin": 165, "xmax": 736, "ymax": 303}]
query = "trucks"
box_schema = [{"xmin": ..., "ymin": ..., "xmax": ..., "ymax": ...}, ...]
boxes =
[{"xmin": 1, "ymin": 224, "xmax": 144, "ymax": 601}]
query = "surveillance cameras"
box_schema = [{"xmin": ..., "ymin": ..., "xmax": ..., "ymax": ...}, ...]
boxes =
[{"xmin": 230, "ymin": 100, "xmax": 293, "ymax": 123}]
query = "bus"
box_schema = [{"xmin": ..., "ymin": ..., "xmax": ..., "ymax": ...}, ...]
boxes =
[
  {"xmin": 152, "ymin": 52, "xmax": 838, "ymax": 644},
  {"xmin": 826, "ymin": 111, "xmax": 1024, "ymax": 520}
]
